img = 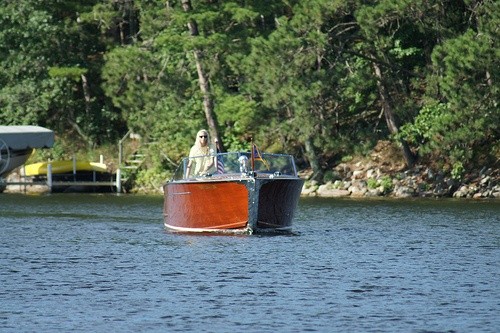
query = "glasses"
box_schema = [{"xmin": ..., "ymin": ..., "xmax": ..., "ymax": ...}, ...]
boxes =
[{"xmin": 199, "ymin": 136, "xmax": 207, "ymax": 138}]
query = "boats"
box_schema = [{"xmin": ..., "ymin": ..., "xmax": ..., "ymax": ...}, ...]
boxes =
[
  {"xmin": 24, "ymin": 161, "xmax": 111, "ymax": 193},
  {"xmin": 161, "ymin": 148, "xmax": 304, "ymax": 237},
  {"xmin": 0, "ymin": 124, "xmax": 55, "ymax": 192}
]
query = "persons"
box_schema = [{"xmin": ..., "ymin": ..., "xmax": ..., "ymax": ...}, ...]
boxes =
[{"xmin": 185, "ymin": 129, "xmax": 217, "ymax": 178}]
